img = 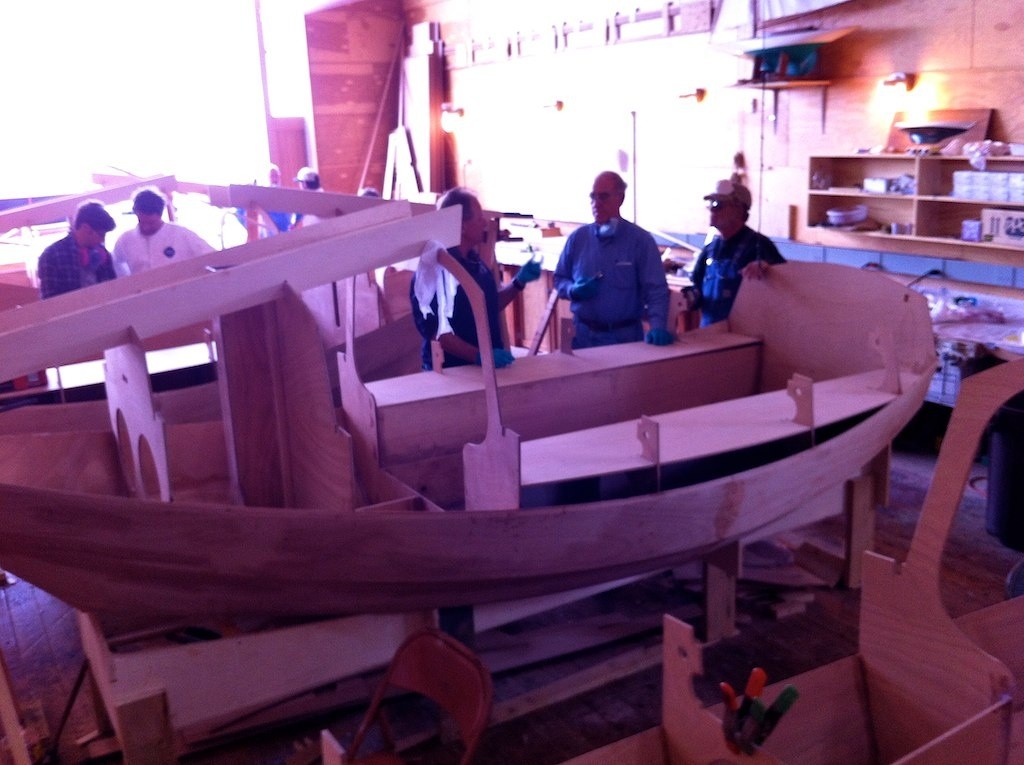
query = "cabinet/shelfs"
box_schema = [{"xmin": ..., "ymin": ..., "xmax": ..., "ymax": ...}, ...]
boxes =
[{"xmin": 804, "ymin": 151, "xmax": 1024, "ymax": 252}]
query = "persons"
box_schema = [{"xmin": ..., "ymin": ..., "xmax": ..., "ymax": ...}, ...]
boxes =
[
  {"xmin": 38, "ymin": 202, "xmax": 116, "ymax": 301},
  {"xmin": 239, "ymin": 164, "xmax": 292, "ymax": 237},
  {"xmin": 553, "ymin": 172, "xmax": 677, "ymax": 350},
  {"xmin": 408, "ymin": 185, "xmax": 543, "ymax": 373},
  {"xmin": 680, "ymin": 179, "xmax": 786, "ymax": 328},
  {"xmin": 294, "ymin": 167, "xmax": 326, "ymax": 228},
  {"xmin": 112, "ymin": 187, "xmax": 218, "ymax": 275}
]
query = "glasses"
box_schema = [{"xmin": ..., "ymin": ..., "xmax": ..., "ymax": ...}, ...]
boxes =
[
  {"xmin": 590, "ymin": 190, "xmax": 624, "ymax": 200},
  {"xmin": 711, "ymin": 201, "xmax": 740, "ymax": 208}
]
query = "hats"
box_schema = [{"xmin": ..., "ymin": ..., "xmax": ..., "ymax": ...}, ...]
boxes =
[
  {"xmin": 292, "ymin": 167, "xmax": 319, "ymax": 183},
  {"xmin": 704, "ymin": 180, "xmax": 751, "ymax": 210}
]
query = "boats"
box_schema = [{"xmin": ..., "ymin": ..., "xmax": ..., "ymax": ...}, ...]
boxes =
[{"xmin": 0, "ymin": 183, "xmax": 941, "ymax": 626}]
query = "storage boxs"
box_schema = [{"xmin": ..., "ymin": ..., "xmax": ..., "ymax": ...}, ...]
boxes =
[
  {"xmin": 981, "ymin": 209, "xmax": 1024, "ymax": 249},
  {"xmin": 960, "ymin": 220, "xmax": 980, "ymax": 244}
]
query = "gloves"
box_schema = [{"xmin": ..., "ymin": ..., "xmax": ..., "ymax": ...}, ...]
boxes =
[
  {"xmin": 517, "ymin": 261, "xmax": 542, "ymax": 284},
  {"xmin": 476, "ymin": 347, "xmax": 515, "ymax": 369},
  {"xmin": 571, "ymin": 276, "xmax": 601, "ymax": 301},
  {"xmin": 645, "ymin": 328, "xmax": 673, "ymax": 346}
]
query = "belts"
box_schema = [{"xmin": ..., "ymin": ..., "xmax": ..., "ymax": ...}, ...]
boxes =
[{"xmin": 582, "ymin": 319, "xmax": 637, "ymax": 331}]
